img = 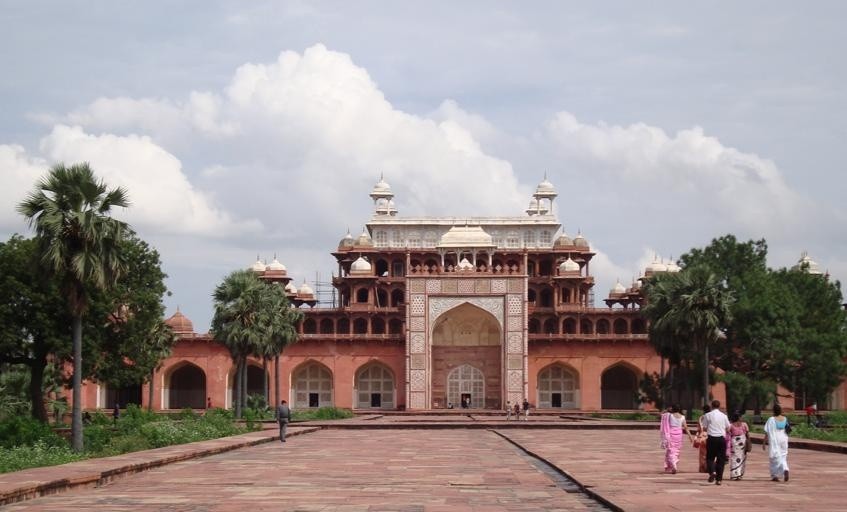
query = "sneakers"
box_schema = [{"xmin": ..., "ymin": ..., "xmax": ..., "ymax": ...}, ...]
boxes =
[
  {"xmin": 773, "ymin": 471, "xmax": 789, "ymax": 481},
  {"xmin": 708, "ymin": 472, "xmax": 721, "ymax": 485}
]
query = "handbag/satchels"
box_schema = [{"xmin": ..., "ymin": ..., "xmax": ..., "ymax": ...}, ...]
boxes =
[{"xmin": 785, "ymin": 424, "xmax": 791, "ymax": 434}]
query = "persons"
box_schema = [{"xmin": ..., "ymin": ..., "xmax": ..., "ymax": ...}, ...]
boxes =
[
  {"xmin": 513, "ymin": 402, "xmax": 521, "ymax": 419},
  {"xmin": 762, "ymin": 404, "xmax": 791, "ymax": 482},
  {"xmin": 660, "ymin": 399, "xmax": 752, "ymax": 485},
  {"xmin": 84, "ymin": 410, "xmax": 91, "ymax": 421},
  {"xmin": 277, "ymin": 400, "xmax": 291, "ymax": 442},
  {"xmin": 523, "ymin": 398, "xmax": 530, "ymax": 420},
  {"xmin": 806, "ymin": 402, "xmax": 817, "ymax": 427},
  {"xmin": 207, "ymin": 396, "xmax": 213, "ymax": 409},
  {"xmin": 113, "ymin": 399, "xmax": 120, "ymax": 428},
  {"xmin": 461, "ymin": 394, "xmax": 471, "ymax": 408},
  {"xmin": 504, "ymin": 401, "xmax": 513, "ymax": 420},
  {"xmin": 447, "ymin": 403, "xmax": 454, "ymax": 409}
]
